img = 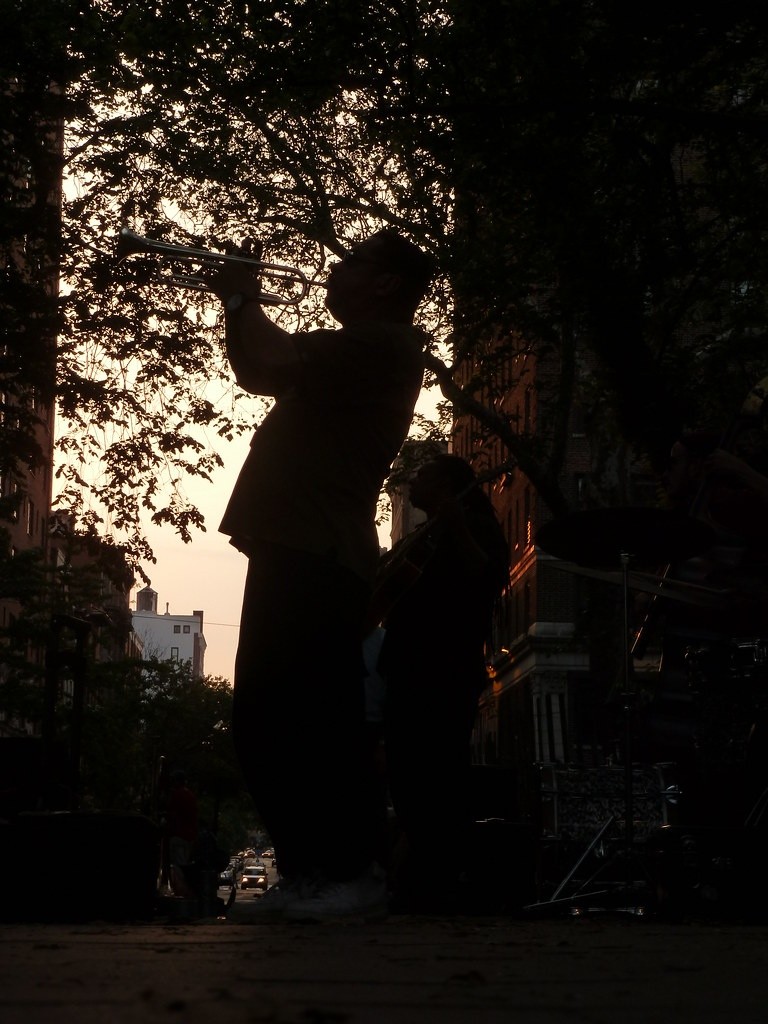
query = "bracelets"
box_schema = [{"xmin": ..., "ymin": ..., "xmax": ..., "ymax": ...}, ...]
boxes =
[{"xmin": 226, "ymin": 288, "xmax": 254, "ymax": 311}]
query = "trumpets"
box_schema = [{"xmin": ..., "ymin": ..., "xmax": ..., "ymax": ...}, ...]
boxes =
[{"xmin": 111, "ymin": 222, "xmax": 326, "ymax": 310}]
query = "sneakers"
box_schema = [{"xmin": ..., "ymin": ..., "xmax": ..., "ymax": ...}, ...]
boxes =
[
  {"xmin": 281, "ymin": 881, "xmax": 388, "ymax": 920},
  {"xmin": 225, "ymin": 879, "xmax": 321, "ymax": 924}
]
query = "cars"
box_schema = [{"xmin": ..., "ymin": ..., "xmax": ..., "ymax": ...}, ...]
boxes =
[
  {"xmin": 215, "ymin": 848, "xmax": 256, "ymax": 889},
  {"xmin": 259, "ymin": 847, "xmax": 277, "ymax": 867},
  {"xmin": 241, "ymin": 867, "xmax": 268, "ymax": 890}
]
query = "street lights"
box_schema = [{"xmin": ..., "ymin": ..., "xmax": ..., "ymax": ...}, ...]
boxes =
[
  {"xmin": 44, "ymin": 615, "xmax": 91, "ymax": 750},
  {"xmin": 609, "ymin": 505, "xmax": 645, "ymax": 892},
  {"xmin": 69, "ymin": 605, "xmax": 112, "ymax": 810}
]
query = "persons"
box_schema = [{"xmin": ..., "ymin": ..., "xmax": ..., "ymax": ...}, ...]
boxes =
[
  {"xmin": 199, "ymin": 233, "xmax": 438, "ymax": 920},
  {"xmin": 378, "ymin": 454, "xmax": 509, "ymax": 914},
  {"xmin": 164, "ymin": 770, "xmax": 200, "ymax": 926}
]
